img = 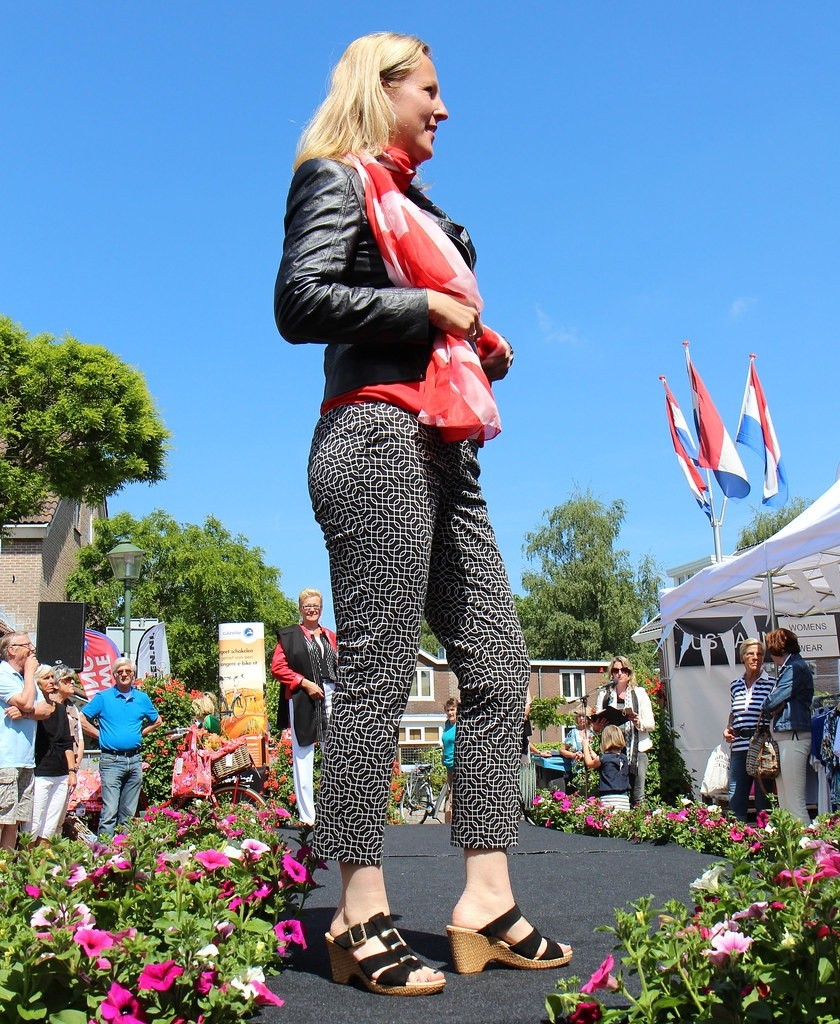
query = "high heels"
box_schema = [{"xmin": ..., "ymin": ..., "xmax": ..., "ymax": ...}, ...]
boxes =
[
  {"xmin": 324, "ymin": 913, "xmax": 447, "ymax": 995},
  {"xmin": 447, "ymin": 901, "xmax": 572, "ymax": 973}
]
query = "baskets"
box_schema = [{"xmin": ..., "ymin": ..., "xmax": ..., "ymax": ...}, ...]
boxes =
[{"xmin": 213, "ymin": 744, "xmax": 261, "ymax": 782}]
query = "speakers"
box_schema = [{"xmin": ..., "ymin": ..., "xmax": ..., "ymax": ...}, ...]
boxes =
[{"xmin": 34, "ymin": 602, "xmax": 86, "ymax": 674}]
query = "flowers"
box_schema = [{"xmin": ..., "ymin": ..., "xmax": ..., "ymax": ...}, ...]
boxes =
[{"xmin": 198, "ymin": 728, "xmax": 232, "ymax": 754}]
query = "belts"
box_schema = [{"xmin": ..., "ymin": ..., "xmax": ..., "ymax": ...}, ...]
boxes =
[{"xmin": 101, "ymin": 748, "xmax": 141, "ymax": 758}]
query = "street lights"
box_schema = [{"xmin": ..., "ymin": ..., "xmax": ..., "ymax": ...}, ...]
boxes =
[{"xmin": 104, "ymin": 536, "xmax": 146, "ymax": 660}]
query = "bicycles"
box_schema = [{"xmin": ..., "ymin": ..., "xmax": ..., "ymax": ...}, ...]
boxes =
[
  {"xmin": 130, "ymin": 726, "xmax": 272, "ymax": 849},
  {"xmin": 399, "ymin": 762, "xmax": 451, "ymax": 825}
]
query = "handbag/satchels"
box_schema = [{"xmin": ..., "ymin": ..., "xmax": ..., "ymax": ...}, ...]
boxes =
[
  {"xmin": 745, "ymin": 709, "xmax": 780, "ymax": 779},
  {"xmin": 171, "ymin": 725, "xmax": 211, "ymax": 798},
  {"xmin": 701, "ymin": 735, "xmax": 732, "ymax": 801}
]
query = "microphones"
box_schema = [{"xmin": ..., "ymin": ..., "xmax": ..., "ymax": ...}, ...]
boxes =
[{"xmin": 599, "ymin": 679, "xmax": 618, "ymax": 688}]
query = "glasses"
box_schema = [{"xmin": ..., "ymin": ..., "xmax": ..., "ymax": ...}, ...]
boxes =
[
  {"xmin": 114, "ymin": 670, "xmax": 133, "ymax": 677},
  {"xmin": 56, "ymin": 675, "xmax": 75, "ymax": 685},
  {"xmin": 301, "ymin": 604, "xmax": 321, "ymax": 610},
  {"xmin": 6, "ymin": 641, "xmax": 34, "ymax": 649},
  {"xmin": 611, "ymin": 667, "xmax": 630, "ymax": 673}
]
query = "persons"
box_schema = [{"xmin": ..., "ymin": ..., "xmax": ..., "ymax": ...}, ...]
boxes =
[
  {"xmin": 49, "ymin": 664, "xmax": 84, "ymax": 834},
  {"xmin": 273, "ymin": 30, "xmax": 573, "ymax": 997},
  {"xmin": 271, "ymin": 589, "xmax": 338, "ymax": 826},
  {"xmin": 0, "ymin": 630, "xmax": 51, "ymax": 863},
  {"xmin": 595, "ymin": 656, "xmax": 655, "ymax": 810},
  {"xmin": 724, "ymin": 638, "xmax": 778, "ymax": 821},
  {"xmin": 525, "ymin": 692, "xmax": 552, "ymax": 757},
  {"xmin": 582, "ymin": 724, "xmax": 632, "ymax": 814},
  {"xmin": 559, "ymin": 709, "xmax": 595, "ymax": 796},
  {"xmin": 79, "ymin": 657, "xmax": 162, "ymax": 839},
  {"xmin": 442, "ymin": 697, "xmax": 460, "ymax": 824},
  {"xmin": 20, "ymin": 664, "xmax": 78, "ymax": 855},
  {"xmin": 763, "ymin": 627, "xmax": 814, "ymax": 827}
]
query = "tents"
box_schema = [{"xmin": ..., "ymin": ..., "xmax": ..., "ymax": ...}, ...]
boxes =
[{"xmin": 659, "ymin": 478, "xmax": 840, "ymax": 802}]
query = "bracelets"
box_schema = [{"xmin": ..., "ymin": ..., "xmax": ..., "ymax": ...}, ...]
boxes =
[
  {"xmin": 506, "ymin": 354, "xmax": 514, "ymax": 368},
  {"xmin": 68, "ymin": 767, "xmax": 78, "ymax": 773}
]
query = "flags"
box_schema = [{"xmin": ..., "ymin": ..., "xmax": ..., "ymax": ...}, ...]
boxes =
[
  {"xmin": 664, "ymin": 381, "xmax": 713, "ymax": 527},
  {"xmin": 736, "ymin": 360, "xmax": 790, "ymax": 509},
  {"xmin": 687, "ymin": 359, "xmax": 751, "ymax": 504}
]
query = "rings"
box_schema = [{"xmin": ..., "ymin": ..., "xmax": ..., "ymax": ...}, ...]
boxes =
[{"xmin": 469, "ymin": 329, "xmax": 477, "ymax": 338}]
